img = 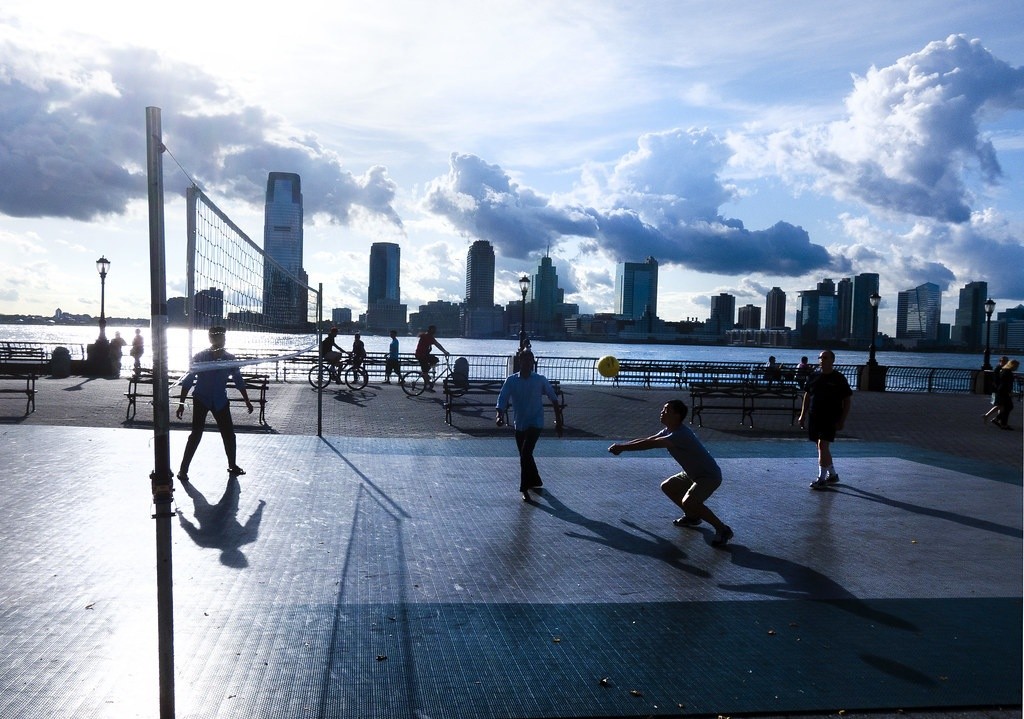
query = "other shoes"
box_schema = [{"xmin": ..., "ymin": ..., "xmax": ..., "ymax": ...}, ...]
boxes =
[
  {"xmin": 990, "ymin": 418, "xmax": 1001, "ymax": 428},
  {"xmin": 525, "ymin": 482, "xmax": 544, "ymax": 489},
  {"xmin": 826, "ymin": 474, "xmax": 839, "ymax": 483},
  {"xmin": 672, "ymin": 514, "xmax": 702, "ymax": 526},
  {"xmin": 1000, "ymin": 427, "xmax": 1014, "ymax": 431},
  {"xmin": 982, "ymin": 414, "xmax": 987, "ymax": 424},
  {"xmin": 711, "ymin": 525, "xmax": 733, "ymax": 549},
  {"xmin": 810, "ymin": 479, "xmax": 827, "ymax": 487},
  {"xmin": 522, "ymin": 491, "xmax": 530, "ymax": 503},
  {"xmin": 335, "ymin": 380, "xmax": 345, "ymax": 385}
]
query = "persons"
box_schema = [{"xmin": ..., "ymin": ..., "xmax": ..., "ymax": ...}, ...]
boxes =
[
  {"xmin": 415, "ymin": 324, "xmax": 450, "ymax": 385},
  {"xmin": 174, "ymin": 324, "xmax": 254, "ymax": 479},
  {"xmin": 318, "ymin": 327, "xmax": 351, "ymax": 385},
  {"xmin": 381, "ymin": 330, "xmax": 406, "ymax": 385},
  {"xmin": 763, "ymin": 355, "xmax": 784, "ymax": 376},
  {"xmin": 109, "ymin": 331, "xmax": 128, "ymax": 363},
  {"xmin": 130, "ymin": 329, "xmax": 144, "ymax": 367},
  {"xmin": 793, "ymin": 356, "xmax": 814, "ymax": 390},
  {"xmin": 798, "ymin": 348, "xmax": 854, "ymax": 488},
  {"xmin": 981, "ymin": 355, "xmax": 1020, "ymax": 429},
  {"xmin": 517, "ymin": 330, "xmax": 535, "ymax": 370},
  {"xmin": 350, "ymin": 334, "xmax": 366, "ymax": 384},
  {"xmin": 606, "ymin": 399, "xmax": 733, "ymax": 547},
  {"xmin": 495, "ymin": 349, "xmax": 560, "ymax": 500}
]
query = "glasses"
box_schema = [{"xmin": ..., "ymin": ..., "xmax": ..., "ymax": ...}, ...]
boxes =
[{"xmin": 818, "ymin": 355, "xmax": 830, "ymax": 360}]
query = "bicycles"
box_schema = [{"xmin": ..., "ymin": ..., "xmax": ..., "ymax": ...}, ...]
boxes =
[
  {"xmin": 309, "ymin": 354, "xmax": 369, "ymax": 390},
  {"xmin": 401, "ymin": 351, "xmax": 471, "ymax": 397}
]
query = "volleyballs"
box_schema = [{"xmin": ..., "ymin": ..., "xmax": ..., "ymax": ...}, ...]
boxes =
[{"xmin": 596, "ymin": 354, "xmax": 621, "ymax": 379}]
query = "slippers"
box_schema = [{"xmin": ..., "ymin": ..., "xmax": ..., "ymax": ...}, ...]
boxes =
[
  {"xmin": 228, "ymin": 466, "xmax": 246, "ymax": 474},
  {"xmin": 177, "ymin": 473, "xmax": 190, "ymax": 480}
]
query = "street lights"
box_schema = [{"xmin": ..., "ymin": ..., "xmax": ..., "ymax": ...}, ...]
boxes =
[
  {"xmin": 866, "ymin": 292, "xmax": 883, "ymax": 366},
  {"xmin": 979, "ymin": 297, "xmax": 995, "ymax": 371},
  {"xmin": 96, "ymin": 255, "xmax": 113, "ymax": 342},
  {"xmin": 517, "ymin": 275, "xmax": 532, "ymax": 351}
]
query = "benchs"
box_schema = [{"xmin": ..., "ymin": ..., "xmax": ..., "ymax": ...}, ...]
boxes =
[
  {"xmin": 689, "ymin": 381, "xmax": 803, "ymax": 430},
  {"xmin": 232, "ymin": 353, "xmax": 278, "ymax": 381},
  {"xmin": 611, "ymin": 361, "xmax": 817, "ymax": 394},
  {"xmin": 0, "ymin": 371, "xmax": 39, "ymax": 415},
  {"xmin": 125, "ymin": 367, "xmax": 268, "ymax": 426},
  {"xmin": 442, "ymin": 377, "xmax": 565, "ymax": 431},
  {"xmin": 0, "ymin": 347, "xmax": 55, "ymax": 372},
  {"xmin": 283, "ymin": 356, "xmax": 438, "ymax": 383}
]
